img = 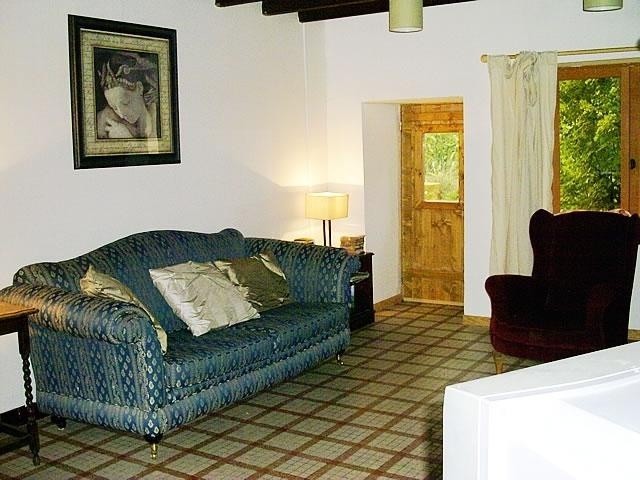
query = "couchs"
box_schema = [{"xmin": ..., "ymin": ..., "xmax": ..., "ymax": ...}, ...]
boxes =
[{"xmin": 0, "ymin": 226, "xmax": 362, "ymax": 462}]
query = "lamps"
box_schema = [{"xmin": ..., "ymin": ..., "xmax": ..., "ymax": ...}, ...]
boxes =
[
  {"xmin": 388, "ymin": 0, "xmax": 424, "ymax": 33},
  {"xmin": 583, "ymin": 0, "xmax": 624, "ymax": 12},
  {"xmin": 304, "ymin": 191, "xmax": 351, "ymax": 248}
]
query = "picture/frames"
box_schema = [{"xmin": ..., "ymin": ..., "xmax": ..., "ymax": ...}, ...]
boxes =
[{"xmin": 67, "ymin": 13, "xmax": 181, "ymax": 171}]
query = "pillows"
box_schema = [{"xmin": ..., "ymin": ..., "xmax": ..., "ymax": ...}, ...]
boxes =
[
  {"xmin": 77, "ymin": 262, "xmax": 168, "ymax": 356},
  {"xmin": 147, "ymin": 260, "xmax": 262, "ymax": 338},
  {"xmin": 207, "ymin": 243, "xmax": 298, "ymax": 314}
]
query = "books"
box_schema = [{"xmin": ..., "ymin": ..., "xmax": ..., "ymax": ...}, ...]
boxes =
[{"xmin": 349, "ymin": 271, "xmax": 370, "ymax": 283}]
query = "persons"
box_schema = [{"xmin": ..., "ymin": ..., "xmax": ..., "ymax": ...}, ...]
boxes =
[{"xmin": 95, "ymin": 47, "xmax": 158, "ymax": 137}]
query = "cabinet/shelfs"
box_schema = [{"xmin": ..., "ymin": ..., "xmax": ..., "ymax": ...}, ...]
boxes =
[{"xmin": 343, "ymin": 248, "xmax": 377, "ymax": 333}]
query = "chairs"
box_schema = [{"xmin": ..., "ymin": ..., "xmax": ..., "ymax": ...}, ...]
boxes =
[{"xmin": 482, "ymin": 205, "xmax": 639, "ymax": 373}]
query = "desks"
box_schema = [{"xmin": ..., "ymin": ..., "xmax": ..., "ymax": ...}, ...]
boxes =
[
  {"xmin": 343, "ymin": 268, "xmax": 370, "ymax": 330},
  {"xmin": 0, "ymin": 295, "xmax": 46, "ymax": 468}
]
